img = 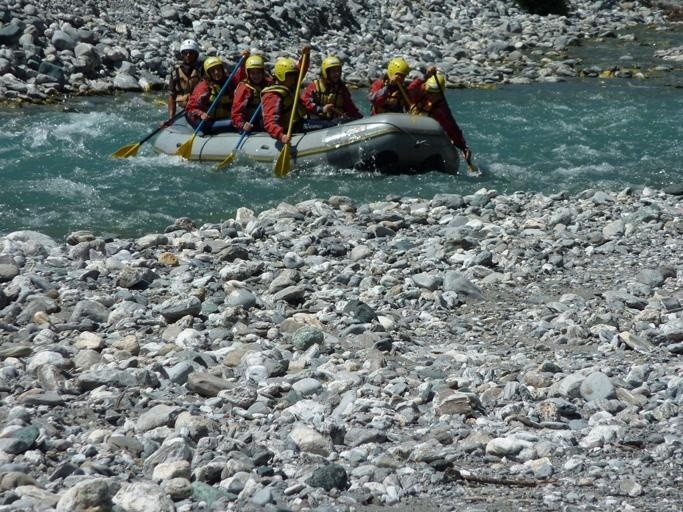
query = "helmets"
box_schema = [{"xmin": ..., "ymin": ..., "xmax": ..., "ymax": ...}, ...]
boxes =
[
  {"xmin": 273, "ymin": 57, "xmax": 298, "ymax": 82},
  {"xmin": 386, "ymin": 57, "xmax": 410, "ymax": 80},
  {"xmin": 426, "ymin": 72, "xmax": 446, "ymax": 93},
  {"xmin": 321, "ymin": 57, "xmax": 344, "ymax": 79},
  {"xmin": 203, "ymin": 56, "xmax": 223, "ymax": 77},
  {"xmin": 244, "ymin": 55, "xmax": 265, "ymax": 78},
  {"xmin": 179, "ymin": 39, "xmax": 200, "ymax": 54}
]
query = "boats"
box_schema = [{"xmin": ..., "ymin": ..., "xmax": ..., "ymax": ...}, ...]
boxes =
[{"xmin": 153, "ymin": 112, "xmax": 461, "ymax": 176}]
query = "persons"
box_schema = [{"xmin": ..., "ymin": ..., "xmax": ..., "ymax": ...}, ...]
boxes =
[
  {"xmin": 298, "ymin": 56, "xmax": 363, "ymax": 119},
  {"xmin": 231, "ymin": 54, "xmax": 274, "ymax": 132},
  {"xmin": 260, "ymin": 44, "xmax": 311, "ymax": 144},
  {"xmin": 404, "ymin": 66, "xmax": 471, "ymax": 160},
  {"xmin": 184, "ymin": 49, "xmax": 251, "ymax": 122},
  {"xmin": 159, "ymin": 39, "xmax": 205, "ymax": 129},
  {"xmin": 367, "ymin": 57, "xmax": 410, "ymax": 116}
]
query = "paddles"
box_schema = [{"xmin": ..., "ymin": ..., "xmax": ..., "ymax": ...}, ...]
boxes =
[
  {"xmin": 175, "ymin": 51, "xmax": 247, "ymax": 162},
  {"xmin": 433, "ymin": 71, "xmax": 480, "ymax": 173},
  {"xmin": 110, "ymin": 107, "xmax": 185, "ymax": 158},
  {"xmin": 217, "ymin": 101, "xmax": 263, "ymax": 169},
  {"xmin": 274, "ymin": 51, "xmax": 309, "ymax": 177}
]
